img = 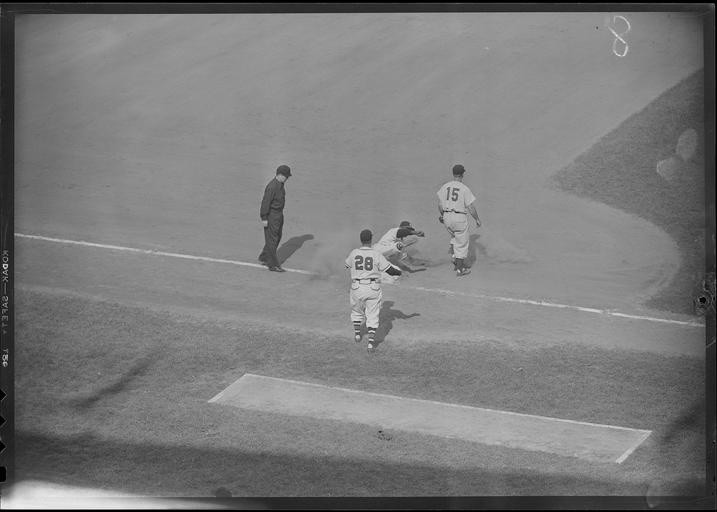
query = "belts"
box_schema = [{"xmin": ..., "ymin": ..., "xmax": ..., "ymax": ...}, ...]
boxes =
[{"xmin": 355, "ymin": 278, "xmax": 378, "ymax": 282}]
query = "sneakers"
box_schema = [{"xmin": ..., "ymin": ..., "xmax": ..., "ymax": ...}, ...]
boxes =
[
  {"xmin": 356, "ymin": 334, "xmax": 361, "ymax": 342},
  {"xmin": 268, "ymin": 265, "xmax": 286, "ymax": 272},
  {"xmin": 455, "ymin": 266, "xmax": 470, "ymax": 277},
  {"xmin": 367, "ymin": 342, "xmax": 374, "ymax": 353}
]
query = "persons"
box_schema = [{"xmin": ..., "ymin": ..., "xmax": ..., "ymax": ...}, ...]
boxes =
[
  {"xmin": 436, "ymin": 164, "xmax": 481, "ymax": 276},
  {"xmin": 257, "ymin": 165, "xmax": 291, "ymax": 272},
  {"xmin": 373, "ymin": 222, "xmax": 427, "ymax": 273},
  {"xmin": 344, "ymin": 230, "xmax": 410, "ymax": 353}
]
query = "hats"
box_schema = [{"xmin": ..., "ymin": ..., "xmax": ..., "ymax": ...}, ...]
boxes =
[
  {"xmin": 399, "ymin": 221, "xmax": 414, "ymax": 230},
  {"xmin": 359, "ymin": 229, "xmax": 372, "ymax": 242},
  {"xmin": 452, "ymin": 165, "xmax": 466, "ymax": 176},
  {"xmin": 276, "ymin": 165, "xmax": 291, "ymax": 178}
]
